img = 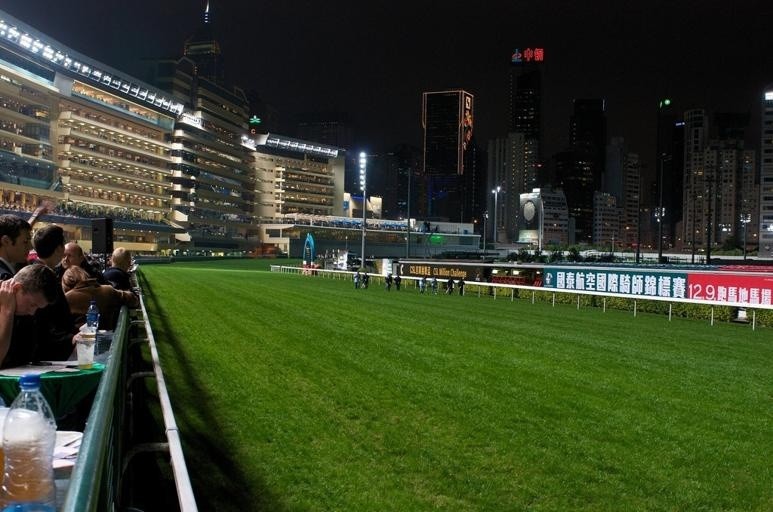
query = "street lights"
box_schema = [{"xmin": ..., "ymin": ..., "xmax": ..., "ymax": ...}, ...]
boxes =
[
  {"xmin": 705, "ymin": 175, "xmax": 715, "ymax": 261},
  {"xmin": 653, "ymin": 205, "xmax": 666, "ymax": 256},
  {"xmin": 490, "ymin": 186, "xmax": 504, "ymax": 244},
  {"xmin": 739, "ymin": 213, "xmax": 752, "ymax": 263},
  {"xmin": 482, "ymin": 210, "xmax": 489, "ymax": 254},
  {"xmin": 345, "ymin": 236, "xmax": 348, "ymax": 249},
  {"xmin": 357, "ymin": 151, "xmax": 370, "ymax": 269}
]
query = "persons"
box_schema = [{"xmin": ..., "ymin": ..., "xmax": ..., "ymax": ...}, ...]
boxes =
[
  {"xmin": 362, "ymin": 272, "xmax": 370, "ymax": 289},
  {"xmin": 394, "ymin": 275, "xmax": 401, "ymax": 290},
  {"xmin": 458, "ymin": 278, "xmax": 465, "ymax": 296},
  {"xmin": 431, "ymin": 277, "xmax": 438, "ymax": 295},
  {"xmin": 354, "ymin": 271, "xmax": 361, "ymax": 289},
  {"xmin": 419, "ymin": 276, "xmax": 426, "ymax": 295},
  {"xmin": 0, "ymin": 215, "xmax": 139, "ymax": 371},
  {"xmin": 385, "ymin": 274, "xmax": 392, "ymax": 292},
  {"xmin": 447, "ymin": 276, "xmax": 455, "ymax": 296}
]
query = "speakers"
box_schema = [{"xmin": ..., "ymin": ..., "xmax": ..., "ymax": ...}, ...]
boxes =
[{"xmin": 92, "ymin": 217, "xmax": 113, "ymax": 253}]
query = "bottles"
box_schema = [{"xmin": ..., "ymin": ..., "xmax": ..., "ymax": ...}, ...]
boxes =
[
  {"xmin": 86, "ymin": 301, "xmax": 98, "ymax": 331},
  {"xmin": 1, "ymin": 374, "xmax": 57, "ymax": 512}
]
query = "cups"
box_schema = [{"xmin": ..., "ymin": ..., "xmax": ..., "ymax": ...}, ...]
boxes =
[
  {"xmin": 74, "ymin": 338, "xmax": 95, "ymax": 368},
  {"xmin": 79, "ymin": 327, "xmax": 96, "ymax": 336}
]
